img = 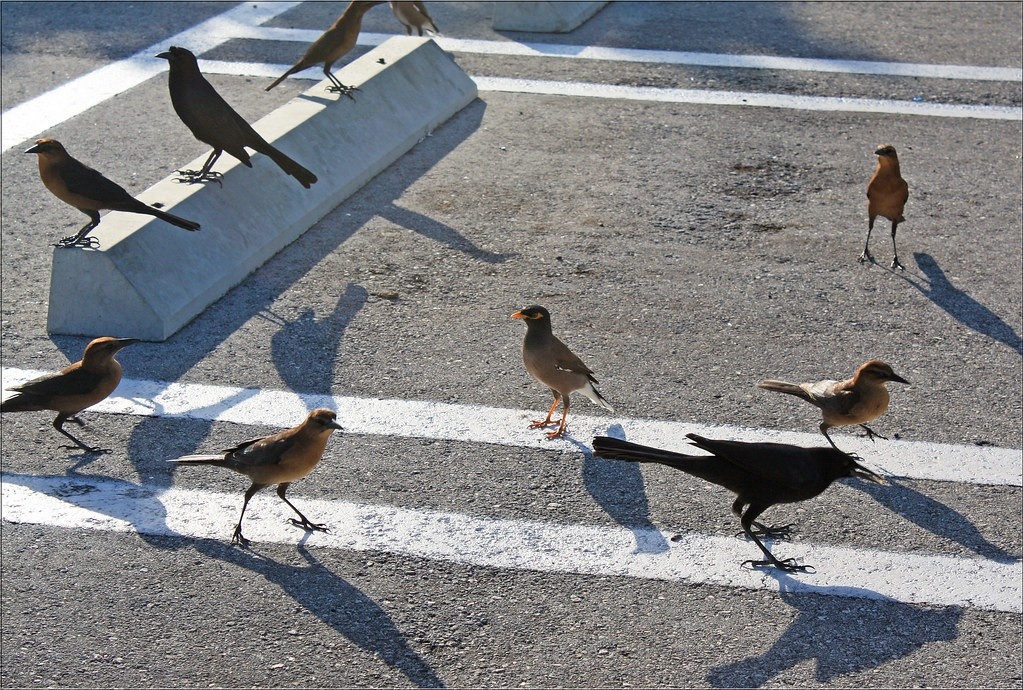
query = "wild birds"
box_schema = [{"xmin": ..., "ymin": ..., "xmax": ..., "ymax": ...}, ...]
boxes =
[
  {"xmin": 856, "ymin": 146, "xmax": 909, "ymax": 267},
  {"xmin": 23, "ymin": 138, "xmax": 201, "ymax": 249},
  {"xmin": 0, "ymin": 337, "xmax": 141, "ymax": 455},
  {"xmin": 264, "ymin": 1, "xmax": 444, "ymax": 106},
  {"xmin": 510, "ymin": 306, "xmax": 611, "ymax": 436},
  {"xmin": 166, "ymin": 408, "xmax": 345, "ymax": 546},
  {"xmin": 757, "ymin": 359, "xmax": 911, "ymax": 462}
]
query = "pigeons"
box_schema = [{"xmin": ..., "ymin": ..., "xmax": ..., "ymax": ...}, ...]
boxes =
[
  {"xmin": 155, "ymin": 45, "xmax": 318, "ymax": 190},
  {"xmin": 591, "ymin": 425, "xmax": 889, "ymax": 574}
]
